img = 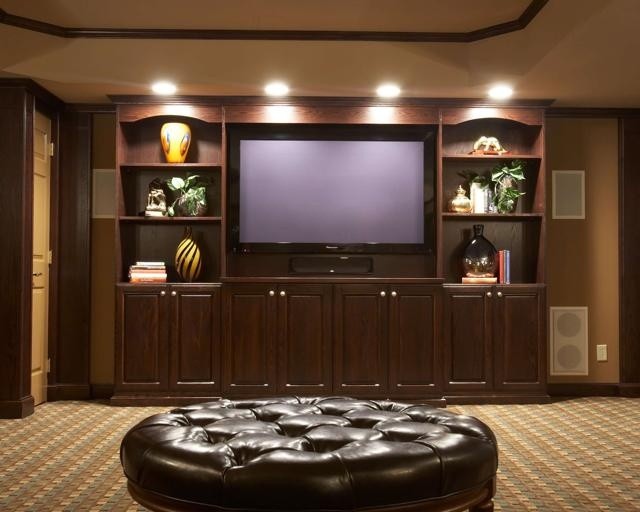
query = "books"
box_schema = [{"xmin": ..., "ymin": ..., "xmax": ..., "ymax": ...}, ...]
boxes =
[
  {"xmin": 129, "ymin": 262, "xmax": 167, "ymax": 283},
  {"xmin": 462, "ymin": 277, "xmax": 497, "ymax": 284},
  {"xmin": 499, "ymin": 250, "xmax": 511, "ymax": 284}
]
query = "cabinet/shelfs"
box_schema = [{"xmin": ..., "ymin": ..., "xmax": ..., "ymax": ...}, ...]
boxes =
[
  {"xmin": 226, "ymin": 93, "xmax": 438, "ymax": 279},
  {"xmin": 115, "ymin": 284, "xmax": 223, "ymax": 393},
  {"xmin": 225, "ymin": 284, "xmax": 336, "ymax": 393},
  {"xmin": 337, "ymin": 285, "xmax": 442, "ymax": 394},
  {"xmin": 114, "ymin": 94, "xmax": 224, "ymax": 284},
  {"xmin": 441, "ymin": 99, "xmax": 546, "ymax": 282},
  {"xmin": 442, "ymin": 286, "xmax": 548, "ymax": 392}
]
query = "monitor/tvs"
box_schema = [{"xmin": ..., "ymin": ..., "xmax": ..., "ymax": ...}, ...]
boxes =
[{"xmin": 228, "ymin": 125, "xmax": 436, "ymax": 255}]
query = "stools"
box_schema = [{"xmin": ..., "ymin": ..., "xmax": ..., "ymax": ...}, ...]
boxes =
[{"xmin": 116, "ymin": 395, "xmax": 500, "ymax": 509}]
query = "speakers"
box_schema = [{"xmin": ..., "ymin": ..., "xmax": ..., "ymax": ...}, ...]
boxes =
[
  {"xmin": 550, "ymin": 306, "xmax": 588, "ymax": 375},
  {"xmin": 92, "ymin": 169, "xmax": 115, "ymax": 218},
  {"xmin": 287, "ymin": 257, "xmax": 373, "ymax": 274},
  {"xmin": 553, "ymin": 170, "xmax": 586, "ymax": 219}
]
